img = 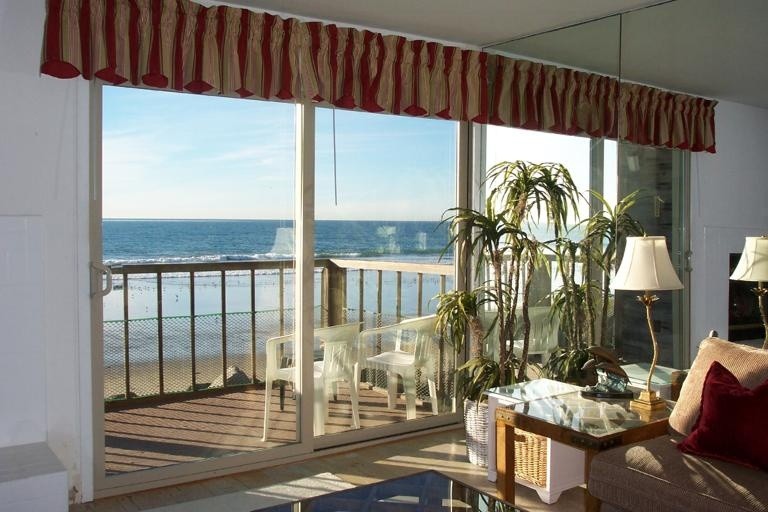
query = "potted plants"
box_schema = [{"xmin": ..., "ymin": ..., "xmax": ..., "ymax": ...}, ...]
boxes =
[{"xmin": 427, "ymin": 159, "xmax": 648, "ymax": 467}]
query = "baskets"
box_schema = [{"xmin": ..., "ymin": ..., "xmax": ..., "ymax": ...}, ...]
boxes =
[
  {"xmin": 464, "ymin": 399, "xmax": 488, "ymax": 467},
  {"xmin": 514, "ymin": 427, "xmax": 547, "ymax": 487}
]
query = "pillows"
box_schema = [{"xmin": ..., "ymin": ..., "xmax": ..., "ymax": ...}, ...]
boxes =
[{"xmin": 676, "ymin": 358, "xmax": 767, "ymax": 472}]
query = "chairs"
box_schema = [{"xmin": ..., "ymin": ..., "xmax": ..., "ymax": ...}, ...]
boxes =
[
  {"xmin": 353, "ymin": 311, "xmax": 439, "ymax": 431},
  {"xmin": 261, "ymin": 318, "xmax": 368, "ymax": 441},
  {"xmin": 586, "ymin": 328, "xmax": 767, "ymax": 511},
  {"xmin": 477, "ymin": 306, "xmax": 561, "ymax": 382}
]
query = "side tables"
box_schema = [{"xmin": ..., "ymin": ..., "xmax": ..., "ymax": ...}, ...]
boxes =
[{"xmin": 493, "ymin": 383, "xmax": 677, "ymax": 503}]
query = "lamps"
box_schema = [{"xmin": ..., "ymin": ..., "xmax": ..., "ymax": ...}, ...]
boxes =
[
  {"xmin": 727, "ymin": 234, "xmax": 768, "ymax": 350},
  {"xmin": 608, "ymin": 230, "xmax": 684, "ymax": 412}
]
